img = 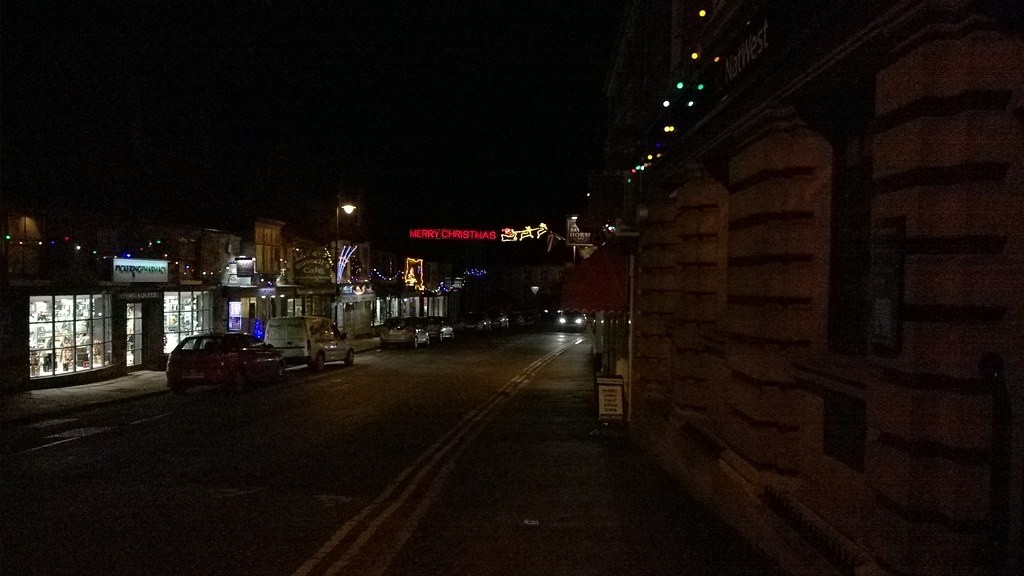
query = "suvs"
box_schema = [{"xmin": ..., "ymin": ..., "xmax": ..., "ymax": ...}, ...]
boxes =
[{"xmin": 262, "ymin": 315, "xmax": 355, "ymax": 374}]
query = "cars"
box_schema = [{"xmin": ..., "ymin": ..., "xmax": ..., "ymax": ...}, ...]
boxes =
[
  {"xmin": 456, "ymin": 306, "xmax": 587, "ymax": 335},
  {"xmin": 166, "ymin": 333, "xmax": 286, "ymax": 396},
  {"xmin": 423, "ymin": 316, "xmax": 454, "ymax": 342},
  {"xmin": 380, "ymin": 315, "xmax": 431, "ymax": 350}
]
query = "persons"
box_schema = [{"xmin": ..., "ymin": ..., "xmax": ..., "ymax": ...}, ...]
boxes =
[
  {"xmin": 59, "ymin": 337, "xmax": 70, "ymax": 371},
  {"xmin": 47, "ymin": 338, "xmax": 52, "ymax": 361}
]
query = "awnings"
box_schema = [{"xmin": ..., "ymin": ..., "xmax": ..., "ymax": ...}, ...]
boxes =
[{"xmin": 560, "ymin": 251, "xmax": 628, "ymax": 314}]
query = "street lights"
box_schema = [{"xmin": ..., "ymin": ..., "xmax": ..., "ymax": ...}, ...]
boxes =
[{"xmin": 334, "ymin": 197, "xmax": 357, "ymax": 329}]
